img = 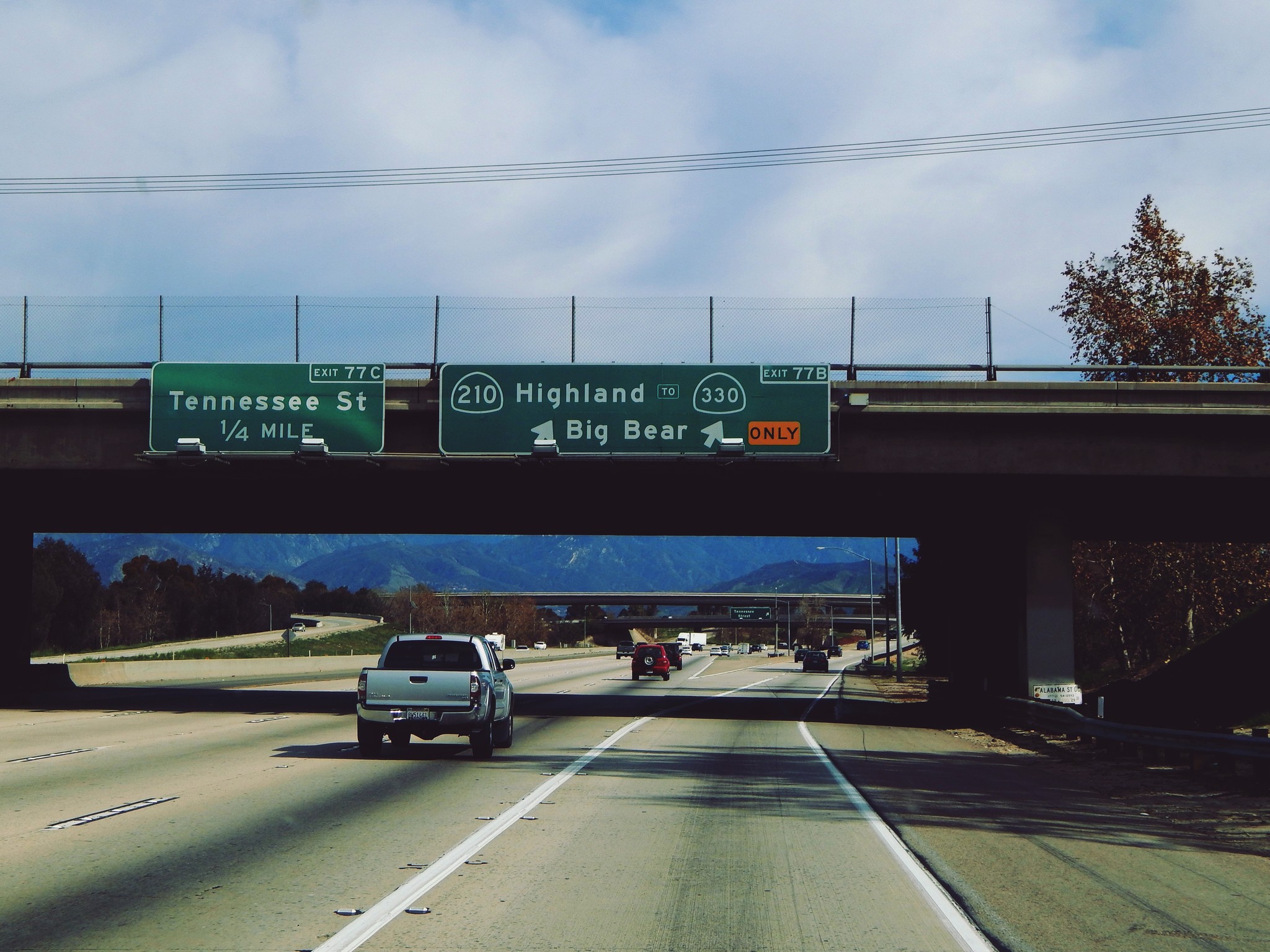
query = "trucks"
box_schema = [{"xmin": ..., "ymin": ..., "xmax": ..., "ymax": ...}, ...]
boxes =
[{"xmin": 675, "ymin": 632, "xmax": 707, "ymax": 647}]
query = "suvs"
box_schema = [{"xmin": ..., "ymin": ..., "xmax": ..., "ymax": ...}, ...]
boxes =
[
  {"xmin": 889, "ymin": 630, "xmax": 897, "ymax": 640},
  {"xmin": 692, "ymin": 643, "xmax": 703, "ymax": 652}
]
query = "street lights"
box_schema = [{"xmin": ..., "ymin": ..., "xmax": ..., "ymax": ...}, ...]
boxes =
[{"xmin": 816, "ymin": 546, "xmax": 874, "ymax": 663}]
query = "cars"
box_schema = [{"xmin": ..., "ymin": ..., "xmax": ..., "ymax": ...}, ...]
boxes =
[
  {"xmin": 802, "ymin": 651, "xmax": 831, "ymax": 673},
  {"xmin": 737, "ymin": 643, "xmax": 768, "ymax": 655},
  {"xmin": 631, "ymin": 644, "xmax": 670, "ymax": 681},
  {"xmin": 794, "ymin": 649, "xmax": 810, "ymax": 663},
  {"xmin": 656, "ymin": 643, "xmax": 682, "ymax": 671},
  {"xmin": 721, "ymin": 648, "xmax": 730, "ymax": 657},
  {"xmin": 636, "ymin": 642, "xmax": 647, "ymax": 648},
  {"xmin": 710, "ymin": 647, "xmax": 722, "ymax": 657},
  {"xmin": 779, "ymin": 643, "xmax": 803, "ymax": 651},
  {"xmin": 827, "ymin": 646, "xmax": 843, "ymax": 657},
  {"xmin": 856, "ymin": 640, "xmax": 870, "ymax": 650},
  {"xmin": 720, "ymin": 643, "xmax": 733, "ymax": 652},
  {"xmin": 680, "ymin": 646, "xmax": 692, "ymax": 656},
  {"xmin": 534, "ymin": 642, "xmax": 547, "ymax": 650},
  {"xmin": 292, "ymin": 623, "xmax": 306, "ymax": 632}
]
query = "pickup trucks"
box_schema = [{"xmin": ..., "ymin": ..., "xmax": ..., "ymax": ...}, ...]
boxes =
[
  {"xmin": 356, "ymin": 633, "xmax": 515, "ymax": 762},
  {"xmin": 616, "ymin": 641, "xmax": 635, "ymax": 660},
  {"xmin": 515, "ymin": 646, "xmax": 529, "ymax": 651}
]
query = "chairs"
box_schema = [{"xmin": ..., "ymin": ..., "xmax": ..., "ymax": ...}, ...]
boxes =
[{"xmin": 396, "ymin": 650, "xmax": 477, "ymax": 667}]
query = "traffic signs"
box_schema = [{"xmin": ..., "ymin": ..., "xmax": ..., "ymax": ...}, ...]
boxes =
[
  {"xmin": 730, "ymin": 607, "xmax": 771, "ymax": 621},
  {"xmin": 437, "ymin": 364, "xmax": 831, "ymax": 460}
]
